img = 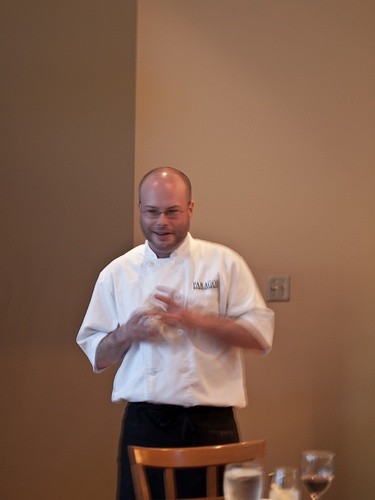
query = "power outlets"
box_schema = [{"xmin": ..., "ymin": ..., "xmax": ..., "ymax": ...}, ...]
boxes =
[{"xmin": 266, "ymin": 275, "xmax": 291, "ymax": 301}]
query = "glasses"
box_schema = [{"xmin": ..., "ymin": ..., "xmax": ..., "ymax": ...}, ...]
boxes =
[{"xmin": 140, "ymin": 203, "xmax": 189, "ymax": 219}]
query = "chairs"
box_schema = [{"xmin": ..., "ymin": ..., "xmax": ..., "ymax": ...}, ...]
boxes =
[{"xmin": 126, "ymin": 439, "xmax": 267, "ymax": 500}]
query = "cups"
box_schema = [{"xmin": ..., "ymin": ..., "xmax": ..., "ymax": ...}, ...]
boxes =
[
  {"xmin": 223, "ymin": 463, "xmax": 263, "ymax": 500},
  {"xmin": 267, "ymin": 465, "xmax": 302, "ymax": 500}
]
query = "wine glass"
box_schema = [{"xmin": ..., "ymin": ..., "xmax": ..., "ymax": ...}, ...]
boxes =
[{"xmin": 300, "ymin": 449, "xmax": 335, "ymax": 500}]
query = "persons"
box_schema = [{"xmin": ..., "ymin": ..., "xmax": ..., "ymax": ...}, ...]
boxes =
[{"xmin": 75, "ymin": 167, "xmax": 276, "ymax": 500}]
